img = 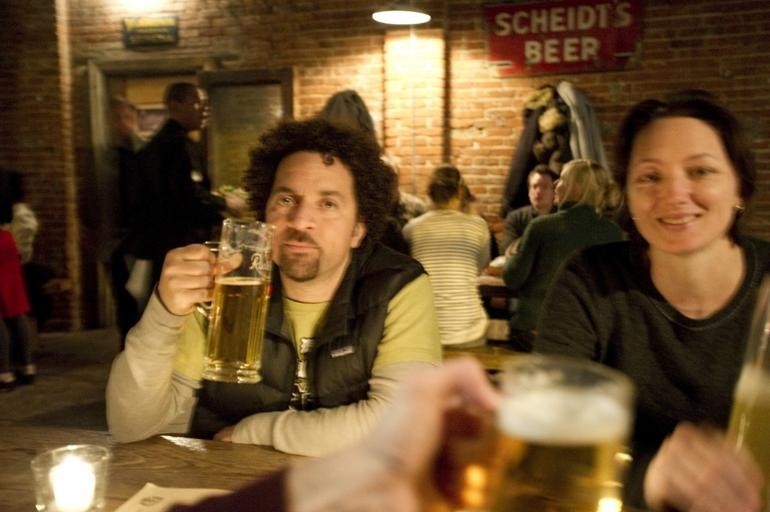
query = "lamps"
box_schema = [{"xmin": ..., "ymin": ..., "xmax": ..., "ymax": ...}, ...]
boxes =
[{"xmin": 371, "ymin": 0, "xmax": 433, "ymax": 26}]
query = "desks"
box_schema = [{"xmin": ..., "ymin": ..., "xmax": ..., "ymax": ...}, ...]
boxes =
[
  {"xmin": 0, "ymin": 418, "xmax": 315, "ymax": 511},
  {"xmin": 477, "ymin": 274, "xmax": 519, "ymax": 319}
]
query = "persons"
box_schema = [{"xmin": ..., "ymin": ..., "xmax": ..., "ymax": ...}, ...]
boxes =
[
  {"xmin": 0, "ymin": 173, "xmax": 46, "ymax": 333},
  {"xmin": 0, "ymin": 193, "xmax": 38, "ymax": 391}
]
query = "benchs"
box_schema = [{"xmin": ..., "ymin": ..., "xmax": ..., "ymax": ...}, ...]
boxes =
[{"xmin": 441, "ymin": 343, "xmax": 535, "ymax": 372}]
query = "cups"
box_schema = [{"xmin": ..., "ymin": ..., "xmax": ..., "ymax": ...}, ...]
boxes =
[
  {"xmin": 28, "ymin": 442, "xmax": 110, "ymax": 512},
  {"xmin": 193, "ymin": 217, "xmax": 277, "ymax": 385},
  {"xmin": 453, "ymin": 350, "xmax": 635, "ymax": 512}
]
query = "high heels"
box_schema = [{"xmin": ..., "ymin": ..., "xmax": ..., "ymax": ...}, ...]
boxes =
[{"xmin": 0, "ymin": 363, "xmax": 37, "ymax": 388}]
